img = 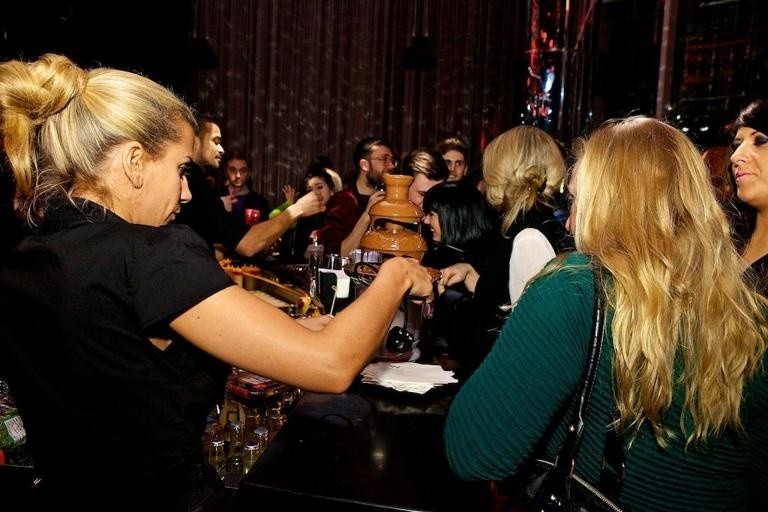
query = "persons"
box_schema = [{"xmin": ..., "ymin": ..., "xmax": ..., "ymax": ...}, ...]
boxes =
[
  {"xmin": 445, "ymin": 116, "xmax": 768, "ymax": 511},
  {"xmin": 0, "ymin": 54, "xmax": 433, "ymax": 510},
  {"xmin": 175, "ymin": 99, "xmax": 768, "ymax": 374}
]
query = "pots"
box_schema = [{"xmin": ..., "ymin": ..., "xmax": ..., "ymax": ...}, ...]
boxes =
[{"xmin": 342, "ymin": 260, "xmax": 443, "ymax": 288}]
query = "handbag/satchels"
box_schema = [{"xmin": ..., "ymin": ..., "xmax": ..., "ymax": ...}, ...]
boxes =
[{"xmin": 510, "ymin": 251, "xmax": 623, "ymax": 512}]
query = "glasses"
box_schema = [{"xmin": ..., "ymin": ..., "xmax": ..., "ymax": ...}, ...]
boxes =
[{"xmin": 367, "ymin": 155, "xmax": 399, "ymax": 167}]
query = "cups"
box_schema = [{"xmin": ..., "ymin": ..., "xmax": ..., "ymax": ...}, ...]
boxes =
[{"xmin": 209, "ymin": 396, "xmax": 287, "ymax": 480}]
